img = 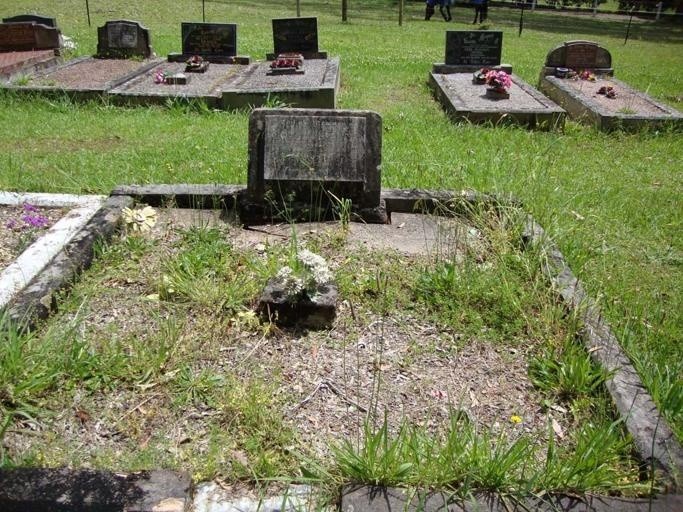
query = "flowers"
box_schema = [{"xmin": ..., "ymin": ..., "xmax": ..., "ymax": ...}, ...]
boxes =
[
  {"xmin": 486, "ymin": 68, "xmax": 511, "ymax": 94},
  {"xmin": 6, "ymin": 200, "xmax": 47, "ymax": 248}
]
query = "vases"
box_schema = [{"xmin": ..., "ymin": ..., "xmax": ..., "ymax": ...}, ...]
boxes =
[{"xmin": 486, "ymin": 89, "xmax": 508, "ymax": 100}]
